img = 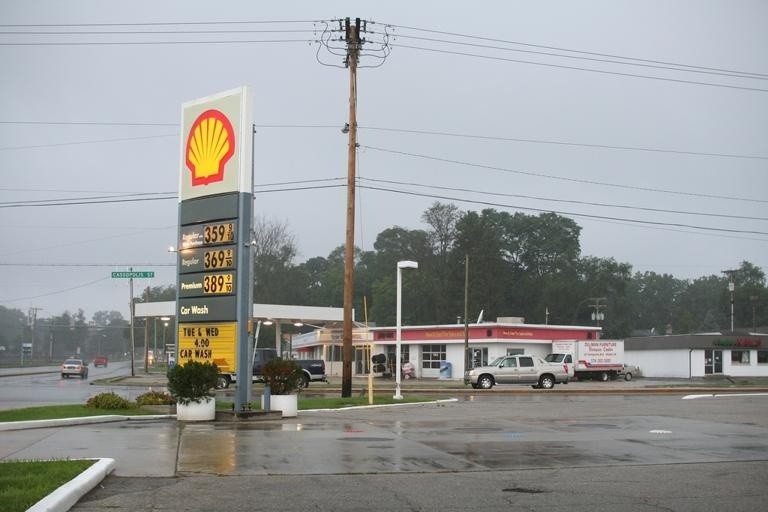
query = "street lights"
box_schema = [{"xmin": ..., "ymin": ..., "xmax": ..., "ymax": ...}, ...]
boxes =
[
  {"xmin": 729, "ymin": 281, "xmax": 735, "ymax": 332},
  {"xmin": 573, "ymin": 297, "xmax": 607, "ymax": 328},
  {"xmin": 393, "ymin": 260, "xmax": 419, "ymax": 399},
  {"xmin": 251, "ymin": 320, "xmax": 273, "ymax": 364}
]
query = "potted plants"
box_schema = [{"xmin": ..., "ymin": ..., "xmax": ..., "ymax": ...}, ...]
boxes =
[
  {"xmin": 167, "ymin": 360, "xmax": 221, "ymax": 422},
  {"xmin": 260, "ymin": 354, "xmax": 308, "ymax": 417}
]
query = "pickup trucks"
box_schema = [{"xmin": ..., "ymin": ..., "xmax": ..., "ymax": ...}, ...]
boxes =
[{"xmin": 215, "ymin": 347, "xmax": 327, "ymax": 390}]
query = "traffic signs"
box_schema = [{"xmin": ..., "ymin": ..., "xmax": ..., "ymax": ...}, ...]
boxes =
[{"xmin": 111, "ymin": 272, "xmax": 155, "ymax": 278}]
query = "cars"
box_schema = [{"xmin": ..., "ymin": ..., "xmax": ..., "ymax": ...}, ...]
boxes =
[
  {"xmin": 463, "ymin": 353, "xmax": 555, "ymax": 388},
  {"xmin": 61, "ymin": 358, "xmax": 89, "ymax": 380},
  {"xmin": 617, "ymin": 364, "xmax": 639, "ymax": 381},
  {"xmin": 93, "ymin": 355, "xmax": 108, "ymax": 367}
]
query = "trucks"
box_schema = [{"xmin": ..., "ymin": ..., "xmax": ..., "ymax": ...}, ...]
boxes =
[{"xmin": 545, "ymin": 340, "xmax": 626, "ymax": 382}]
